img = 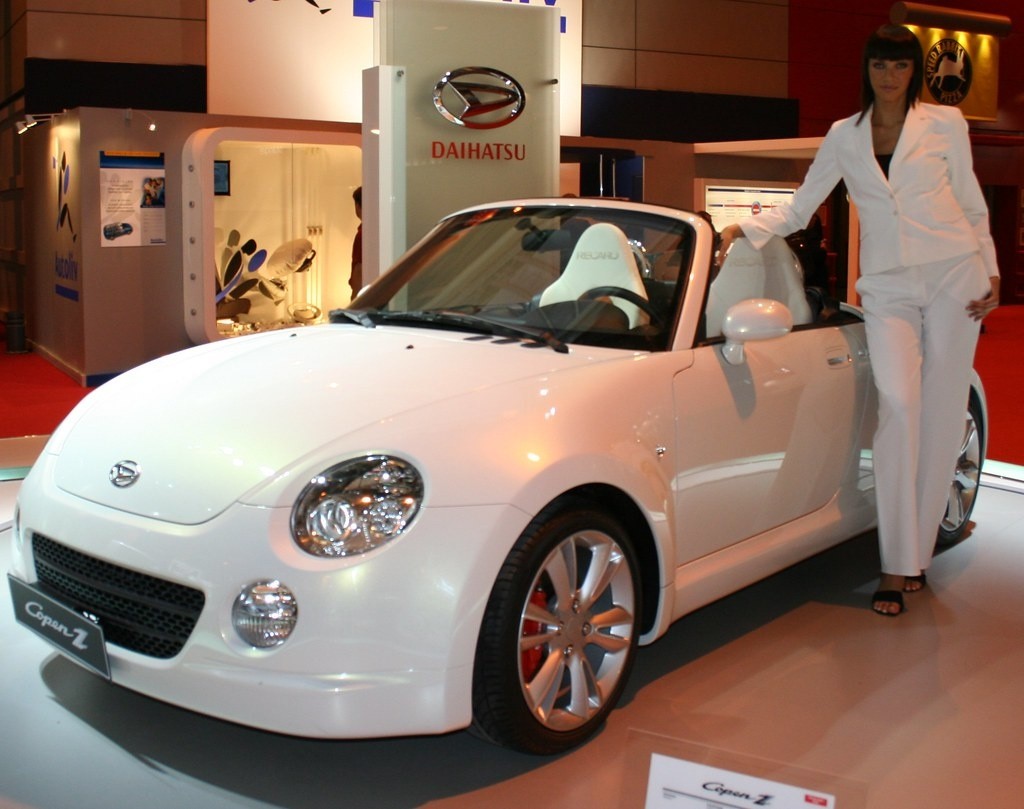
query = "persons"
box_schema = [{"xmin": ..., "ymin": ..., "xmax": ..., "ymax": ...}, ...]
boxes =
[
  {"xmin": 348, "ymin": 186, "xmax": 363, "ymax": 302},
  {"xmin": 559, "ymin": 194, "xmax": 589, "ymax": 275},
  {"xmin": 716, "ymin": 23, "xmax": 1002, "ymax": 615},
  {"xmin": 143, "ymin": 177, "xmax": 165, "ymax": 206}
]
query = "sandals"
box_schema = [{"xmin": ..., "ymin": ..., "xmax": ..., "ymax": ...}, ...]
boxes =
[
  {"xmin": 872, "ymin": 589, "xmax": 904, "ymax": 616},
  {"xmin": 903, "ymin": 573, "xmax": 926, "ymax": 592}
]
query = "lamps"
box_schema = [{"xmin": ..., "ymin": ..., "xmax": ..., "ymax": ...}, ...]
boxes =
[{"xmin": 15, "ymin": 113, "xmax": 62, "ymax": 134}]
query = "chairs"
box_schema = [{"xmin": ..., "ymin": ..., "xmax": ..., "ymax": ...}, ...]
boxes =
[
  {"xmin": 538, "ymin": 223, "xmax": 650, "ymax": 330},
  {"xmin": 705, "ymin": 237, "xmax": 812, "ymax": 339}
]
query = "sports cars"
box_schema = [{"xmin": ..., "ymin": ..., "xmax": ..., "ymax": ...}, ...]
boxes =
[{"xmin": 7, "ymin": 198, "xmax": 988, "ymax": 755}]
